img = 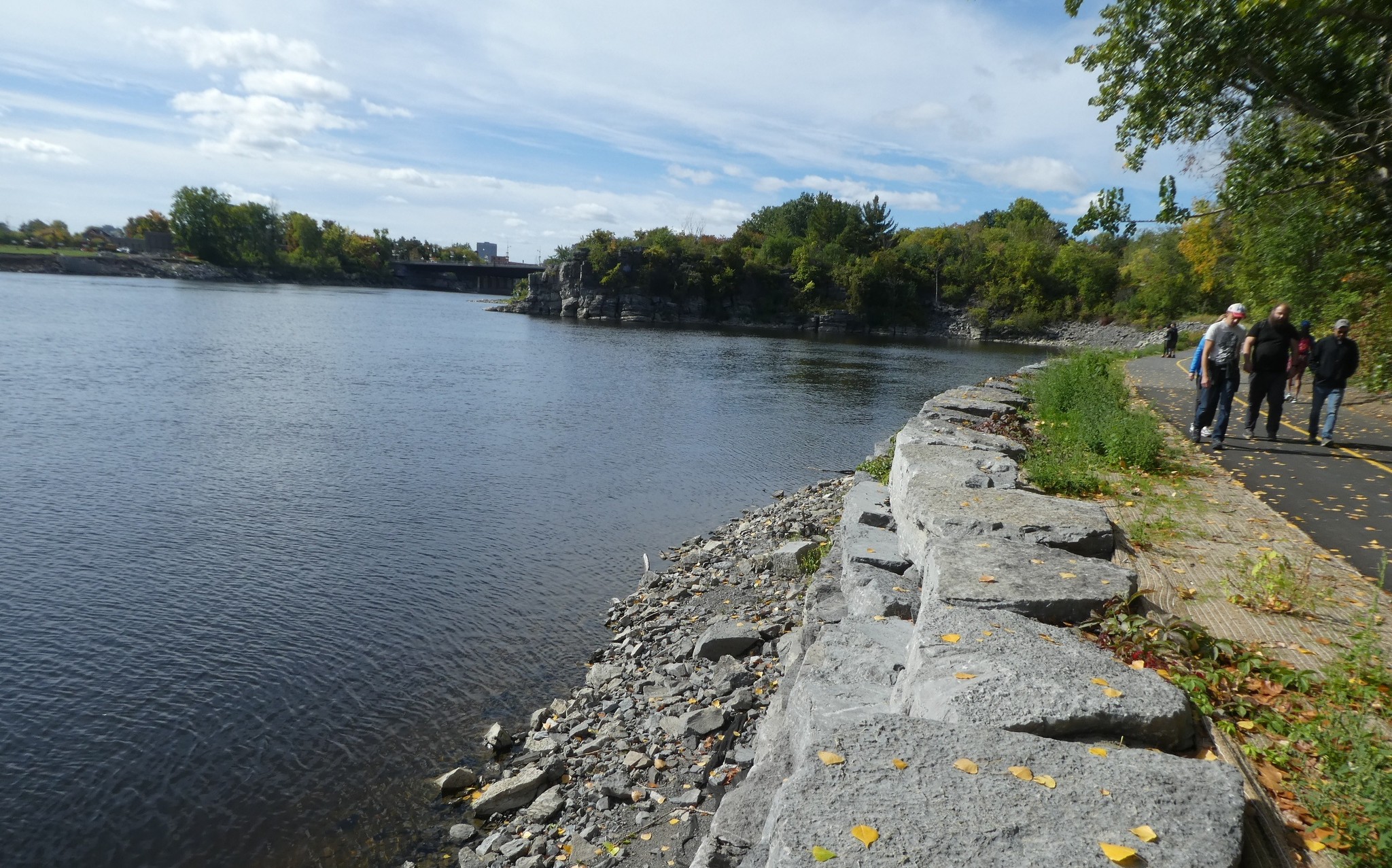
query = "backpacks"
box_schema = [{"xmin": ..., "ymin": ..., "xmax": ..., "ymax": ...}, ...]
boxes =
[{"xmin": 1299, "ymin": 332, "xmax": 1313, "ymax": 355}]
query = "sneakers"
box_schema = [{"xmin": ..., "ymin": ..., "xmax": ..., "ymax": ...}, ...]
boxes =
[
  {"xmin": 1242, "ymin": 428, "xmax": 1254, "ymax": 437},
  {"xmin": 1308, "ymin": 433, "xmax": 1317, "ymax": 442},
  {"xmin": 1289, "ymin": 397, "xmax": 1297, "ymax": 403},
  {"xmin": 1191, "ymin": 427, "xmax": 1202, "ymax": 443},
  {"xmin": 1212, "ymin": 437, "xmax": 1222, "ymax": 450},
  {"xmin": 1201, "ymin": 425, "xmax": 1211, "ymax": 437},
  {"xmin": 1284, "ymin": 393, "xmax": 1292, "ymax": 401},
  {"xmin": 1321, "ymin": 437, "xmax": 1334, "ymax": 446},
  {"xmin": 1267, "ymin": 431, "xmax": 1277, "ymax": 441},
  {"xmin": 1189, "ymin": 423, "xmax": 1195, "ymax": 432}
]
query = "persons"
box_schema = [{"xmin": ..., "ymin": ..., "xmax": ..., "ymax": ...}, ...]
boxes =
[
  {"xmin": 1307, "ymin": 319, "xmax": 1359, "ymax": 446},
  {"xmin": 1191, "ymin": 302, "xmax": 1247, "ymax": 449},
  {"xmin": 1283, "ymin": 320, "xmax": 1316, "ymax": 403},
  {"xmin": 1160, "ymin": 323, "xmax": 1171, "ymax": 357},
  {"xmin": 1243, "ymin": 303, "xmax": 1300, "ymax": 440},
  {"xmin": 1189, "ymin": 313, "xmax": 1228, "ymax": 437},
  {"xmin": 1160, "ymin": 323, "xmax": 1178, "ymax": 358}
]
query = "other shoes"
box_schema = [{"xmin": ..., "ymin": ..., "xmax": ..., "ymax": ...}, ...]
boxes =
[{"xmin": 1161, "ymin": 353, "xmax": 1175, "ymax": 358}]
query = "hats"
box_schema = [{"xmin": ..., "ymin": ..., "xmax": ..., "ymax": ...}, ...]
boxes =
[
  {"xmin": 1335, "ymin": 319, "xmax": 1351, "ymax": 329},
  {"xmin": 1227, "ymin": 303, "xmax": 1247, "ymax": 319}
]
